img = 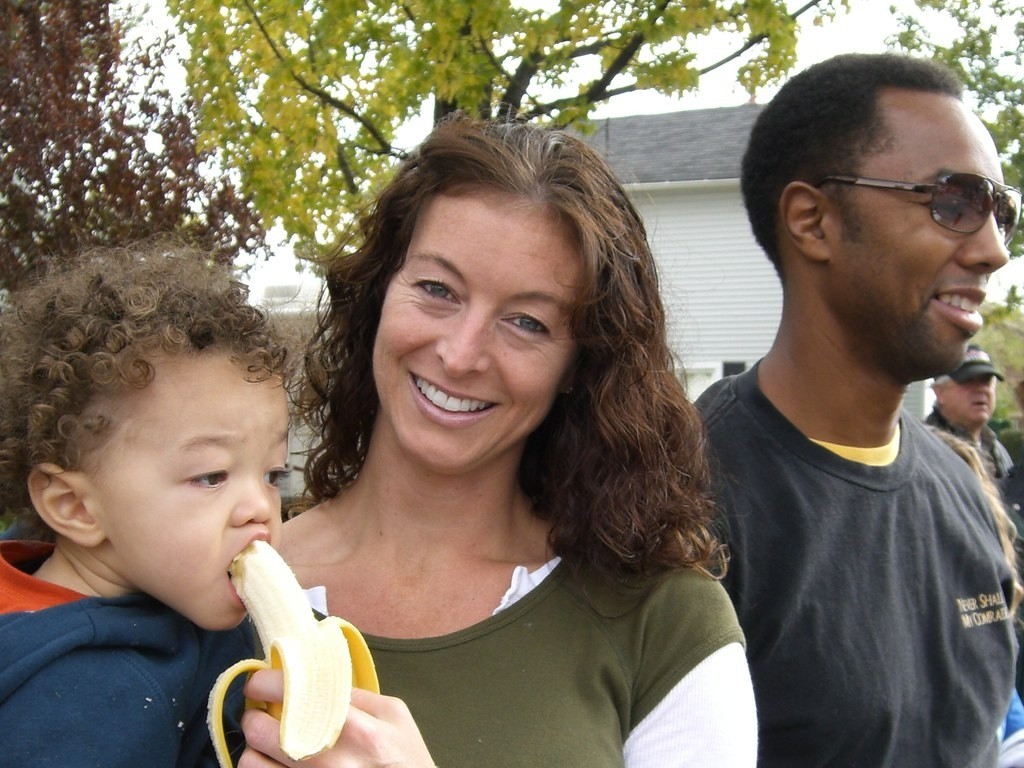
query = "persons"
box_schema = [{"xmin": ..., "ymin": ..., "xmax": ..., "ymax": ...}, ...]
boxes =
[
  {"xmin": 242, "ymin": 120, "xmax": 760, "ymax": 768},
  {"xmin": 0, "ymin": 236, "xmax": 290, "ymax": 768},
  {"xmin": 686, "ymin": 52, "xmax": 1024, "ymax": 768}
]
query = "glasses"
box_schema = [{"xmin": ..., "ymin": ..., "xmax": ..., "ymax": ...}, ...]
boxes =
[{"xmin": 813, "ymin": 171, "xmax": 1022, "ymax": 248}]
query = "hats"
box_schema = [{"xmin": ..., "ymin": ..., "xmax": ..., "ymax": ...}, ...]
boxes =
[{"xmin": 934, "ymin": 344, "xmax": 1005, "ymax": 384}]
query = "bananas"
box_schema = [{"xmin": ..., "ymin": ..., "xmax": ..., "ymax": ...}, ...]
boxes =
[{"xmin": 206, "ymin": 541, "xmax": 379, "ymax": 768}]
